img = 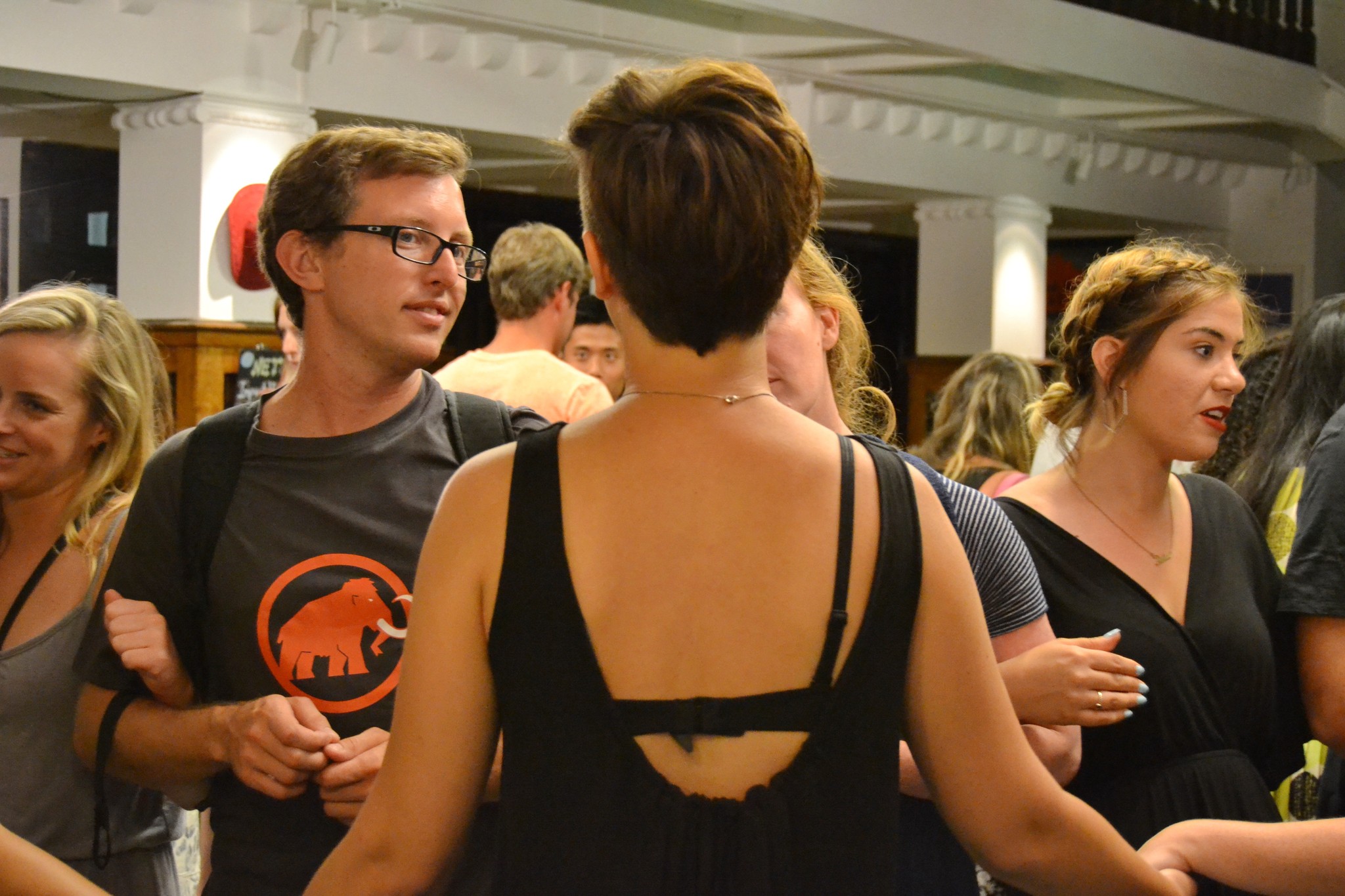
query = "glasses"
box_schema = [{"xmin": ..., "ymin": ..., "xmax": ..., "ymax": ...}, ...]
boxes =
[{"xmin": 291, "ymin": 223, "xmax": 488, "ymax": 282}]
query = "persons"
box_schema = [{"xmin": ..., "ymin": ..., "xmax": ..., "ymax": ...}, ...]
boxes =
[
  {"xmin": 1284, "ymin": 404, "xmax": 1345, "ymax": 756},
  {"xmin": 898, "ymin": 347, "xmax": 1046, "ymax": 497},
  {"xmin": 1232, "ymin": 292, "xmax": 1343, "ymax": 572},
  {"xmin": 562, "ymin": 291, "xmax": 631, "ymax": 405},
  {"xmin": 767, "ymin": 239, "xmax": 1081, "ymax": 789},
  {"xmin": 272, "ymin": 293, "xmax": 307, "ymax": 371},
  {"xmin": 57, "ymin": 118, "xmax": 563, "ymax": 896},
  {"xmin": 1138, "ymin": 808, "xmax": 1345, "ymax": 894},
  {"xmin": 429, "ymin": 222, "xmax": 613, "ymax": 434},
  {"xmin": 292, "ymin": 52, "xmax": 1201, "ymax": 896},
  {"xmin": 986, "ymin": 241, "xmax": 1315, "ymax": 855},
  {"xmin": 0, "ymin": 278, "xmax": 199, "ymax": 892}
]
